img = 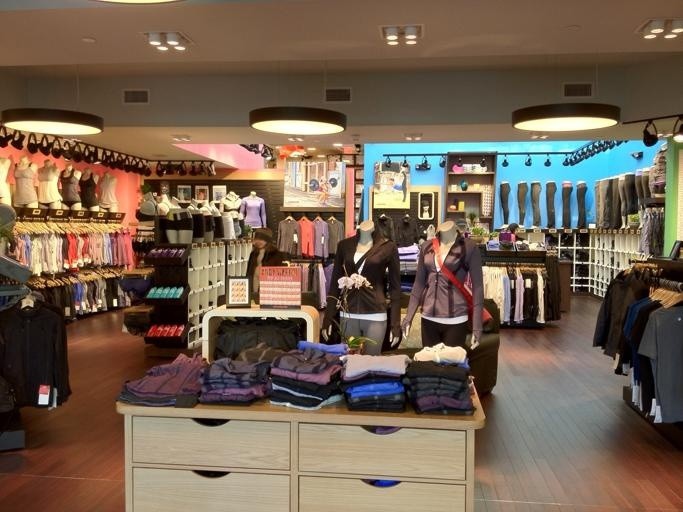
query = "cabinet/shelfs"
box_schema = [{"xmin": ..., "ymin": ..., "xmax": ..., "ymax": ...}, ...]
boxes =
[
  {"xmin": 442, "ymin": 152, "xmax": 497, "ymax": 237},
  {"xmin": 116, "ymin": 374, "xmax": 487, "ymax": 512},
  {"xmin": 144, "ymin": 238, "xmax": 254, "ymax": 357},
  {"xmin": 498, "ymin": 230, "xmax": 644, "ymax": 300}
]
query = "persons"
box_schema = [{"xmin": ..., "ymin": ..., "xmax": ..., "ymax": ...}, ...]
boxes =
[
  {"xmin": 245, "ymin": 227, "xmax": 282, "ymax": 305},
  {"xmin": 509, "ymin": 223, "xmax": 519, "ymax": 242},
  {"xmin": 396, "ymin": 213, "xmax": 421, "ymax": 247},
  {"xmin": 135, "ymin": 191, "xmax": 266, "ymax": 242},
  {"xmin": 375, "ymin": 213, "xmax": 395, "ymax": 242},
  {"xmin": 0, "ymin": 156, "xmax": 119, "ymax": 213},
  {"xmin": 316, "ymin": 176, "xmax": 330, "ymax": 206},
  {"xmin": 322, "ymin": 220, "xmax": 401, "ymax": 355},
  {"xmin": 421, "ymin": 200, "xmax": 432, "ymax": 218},
  {"xmin": 400, "ymin": 166, "xmax": 407, "ymax": 202},
  {"xmin": 0, "ymin": 204, "xmax": 17, "ymax": 254},
  {"xmin": 400, "ymin": 221, "xmax": 484, "ymax": 350}
]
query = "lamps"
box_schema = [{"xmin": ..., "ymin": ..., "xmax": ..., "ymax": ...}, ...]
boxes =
[
  {"xmin": 502, "ymin": 154, "xmax": 569, "ymax": 167},
  {"xmin": 384, "ymin": 156, "xmax": 446, "ymax": 169},
  {"xmin": 1, "ymin": 108, "xmax": 105, "ymax": 135},
  {"xmin": 635, "ymin": 17, "xmax": 682, "ymax": 40},
  {"xmin": 570, "ymin": 139, "xmax": 628, "ymax": 166},
  {"xmin": 385, "ymin": 25, "xmax": 418, "ymax": 47},
  {"xmin": 641, "ymin": 116, "xmax": 683, "ymax": 147},
  {"xmin": 511, "ymin": 103, "xmax": 620, "ymax": 132},
  {"xmin": 149, "ymin": 32, "xmax": 186, "ymax": 52},
  {"xmin": 0, "ymin": 126, "xmax": 216, "ymax": 177},
  {"xmin": 249, "ymin": 106, "xmax": 347, "ymax": 136}
]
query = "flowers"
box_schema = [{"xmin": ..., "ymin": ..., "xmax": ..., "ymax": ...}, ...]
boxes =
[{"xmin": 326, "ymin": 263, "xmax": 378, "ymax": 349}]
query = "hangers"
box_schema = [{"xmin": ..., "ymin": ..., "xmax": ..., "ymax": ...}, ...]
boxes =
[
  {"xmin": 280, "ymin": 213, "xmax": 343, "ymax": 225},
  {"xmin": 12, "ymin": 215, "xmax": 130, "ymax": 235},
  {"xmin": 1, "ymin": 289, "xmax": 63, "ymax": 319},
  {"xmin": 24, "ymin": 265, "xmax": 124, "ymax": 290},
  {"xmin": 482, "ymin": 261, "xmax": 507, "ymax": 279},
  {"xmin": 616, "ymin": 254, "xmax": 683, "ymax": 313}
]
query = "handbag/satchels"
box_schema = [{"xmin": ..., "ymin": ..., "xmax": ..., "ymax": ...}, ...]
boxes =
[
  {"xmin": 213, "ymin": 319, "xmax": 301, "ymax": 360},
  {"xmin": 468, "ymin": 297, "xmax": 492, "ymax": 332}
]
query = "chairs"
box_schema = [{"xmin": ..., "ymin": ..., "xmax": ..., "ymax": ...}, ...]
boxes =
[{"xmin": 462, "ymin": 298, "xmax": 499, "ymax": 398}]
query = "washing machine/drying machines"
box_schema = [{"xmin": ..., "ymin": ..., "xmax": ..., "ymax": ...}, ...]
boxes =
[{"xmin": 327, "ymin": 170, "xmax": 342, "ymax": 200}]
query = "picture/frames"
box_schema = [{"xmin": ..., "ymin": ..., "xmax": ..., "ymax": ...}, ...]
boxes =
[{"xmin": 417, "ymin": 191, "xmax": 435, "ymax": 221}]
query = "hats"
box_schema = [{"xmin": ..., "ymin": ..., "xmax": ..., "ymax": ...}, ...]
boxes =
[{"xmin": 254, "ymin": 228, "xmax": 273, "ymax": 242}]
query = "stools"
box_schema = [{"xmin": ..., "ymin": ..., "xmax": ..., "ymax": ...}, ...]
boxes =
[{"xmin": 123, "ymin": 302, "xmax": 154, "ymax": 337}]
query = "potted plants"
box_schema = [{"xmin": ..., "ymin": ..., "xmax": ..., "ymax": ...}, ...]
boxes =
[
  {"xmin": 467, "ymin": 228, "xmax": 486, "ymax": 245},
  {"xmin": 467, "ymin": 213, "xmax": 476, "ymax": 227}
]
